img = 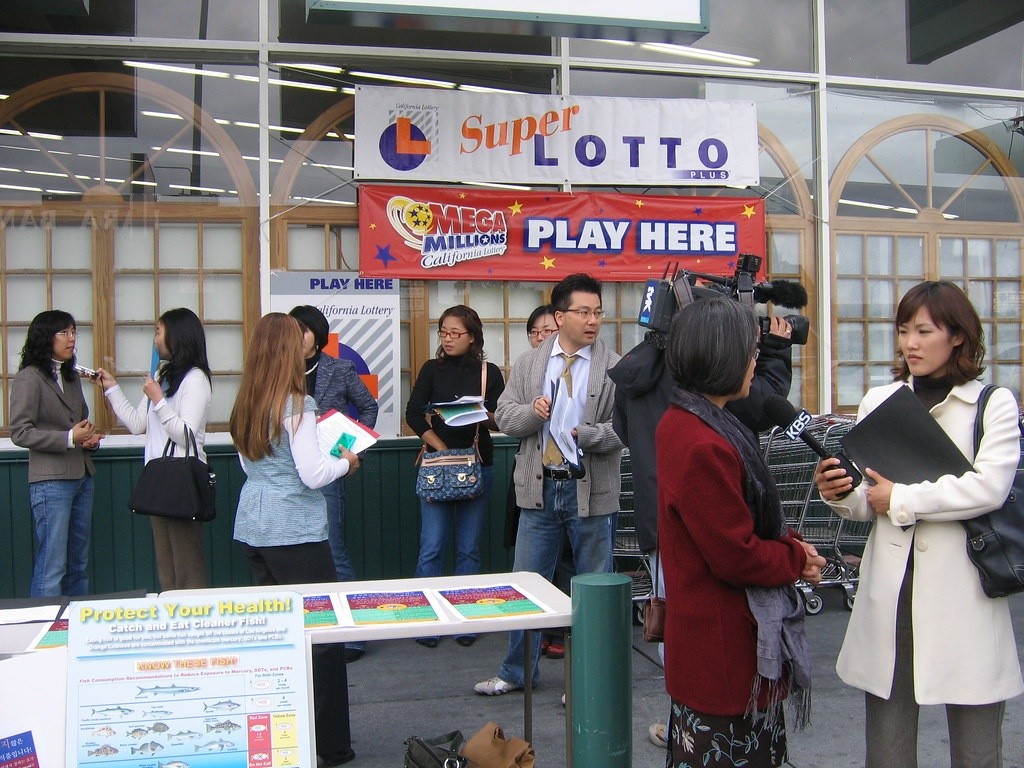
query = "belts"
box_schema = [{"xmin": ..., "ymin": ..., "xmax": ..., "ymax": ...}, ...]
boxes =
[{"xmin": 541, "ymin": 466, "xmax": 586, "ymax": 481}]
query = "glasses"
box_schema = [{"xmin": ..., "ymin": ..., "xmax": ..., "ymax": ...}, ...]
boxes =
[
  {"xmin": 556, "ymin": 308, "xmax": 606, "ymax": 318},
  {"xmin": 752, "ymin": 347, "xmax": 760, "ymax": 360},
  {"xmin": 55, "ymin": 330, "xmax": 79, "ymax": 339},
  {"xmin": 527, "ymin": 328, "xmax": 560, "ymax": 338},
  {"xmin": 437, "ymin": 329, "xmax": 468, "ymax": 338}
]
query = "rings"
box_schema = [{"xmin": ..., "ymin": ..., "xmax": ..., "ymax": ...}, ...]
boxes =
[{"xmin": 786, "ymin": 330, "xmax": 790, "ymax": 334}]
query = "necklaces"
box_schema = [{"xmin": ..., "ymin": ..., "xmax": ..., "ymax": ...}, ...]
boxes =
[{"xmin": 305, "ymin": 361, "xmax": 318, "ymax": 375}]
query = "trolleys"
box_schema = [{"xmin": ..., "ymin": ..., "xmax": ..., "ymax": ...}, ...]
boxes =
[{"xmin": 611, "ymin": 411, "xmax": 876, "ymax": 627}]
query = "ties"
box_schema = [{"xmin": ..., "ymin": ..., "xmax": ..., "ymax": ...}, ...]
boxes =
[{"xmin": 542, "ymin": 352, "xmax": 581, "ymax": 466}]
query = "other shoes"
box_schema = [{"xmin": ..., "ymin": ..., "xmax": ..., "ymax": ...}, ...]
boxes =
[
  {"xmin": 416, "ymin": 638, "xmax": 438, "ymax": 648},
  {"xmin": 456, "ymin": 635, "xmax": 476, "ymax": 646},
  {"xmin": 649, "ymin": 723, "xmax": 668, "ymax": 748},
  {"xmin": 328, "ymin": 750, "xmax": 354, "ymax": 767},
  {"xmin": 345, "ymin": 647, "xmax": 364, "ymax": 664},
  {"xmin": 540, "ymin": 638, "xmax": 564, "ymax": 659}
]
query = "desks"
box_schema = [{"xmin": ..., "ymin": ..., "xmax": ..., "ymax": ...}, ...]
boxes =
[{"xmin": 0, "ymin": 571, "xmax": 571, "ymax": 768}]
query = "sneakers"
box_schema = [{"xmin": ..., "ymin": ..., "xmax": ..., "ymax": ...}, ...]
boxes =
[{"xmin": 473, "ymin": 676, "xmax": 519, "ymax": 695}]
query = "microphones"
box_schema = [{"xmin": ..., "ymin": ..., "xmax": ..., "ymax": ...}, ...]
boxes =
[
  {"xmin": 753, "ymin": 280, "xmax": 808, "ymax": 309},
  {"xmin": 764, "ymin": 395, "xmax": 863, "ymax": 497}
]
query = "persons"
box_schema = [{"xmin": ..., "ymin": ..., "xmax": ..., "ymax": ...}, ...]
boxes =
[
  {"xmin": 656, "ymin": 296, "xmax": 826, "ymax": 768},
  {"xmin": 405, "ymin": 305, "xmax": 508, "ymax": 647},
  {"xmin": 610, "ymin": 315, "xmax": 792, "ymax": 748},
  {"xmin": 229, "ymin": 311, "xmax": 360, "ymax": 587},
  {"xmin": 90, "ymin": 307, "xmax": 213, "ymax": 591},
  {"xmin": 473, "ymin": 273, "xmax": 623, "ymax": 706},
  {"xmin": 9, "ymin": 310, "xmax": 106, "ymax": 598},
  {"xmin": 502, "ymin": 305, "xmax": 578, "ymax": 657},
  {"xmin": 288, "ymin": 305, "xmax": 380, "ymax": 665},
  {"xmin": 816, "ymin": 280, "xmax": 1024, "ymax": 768}
]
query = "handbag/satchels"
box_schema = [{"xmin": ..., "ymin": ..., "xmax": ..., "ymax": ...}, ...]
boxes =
[
  {"xmin": 403, "ymin": 730, "xmax": 468, "ymax": 768},
  {"xmin": 129, "ymin": 424, "xmax": 217, "ymax": 522},
  {"xmin": 960, "ymin": 384, "xmax": 1024, "ymax": 598},
  {"xmin": 642, "ymin": 595, "xmax": 666, "ymax": 642},
  {"xmin": 415, "ymin": 446, "xmax": 484, "ymax": 503}
]
